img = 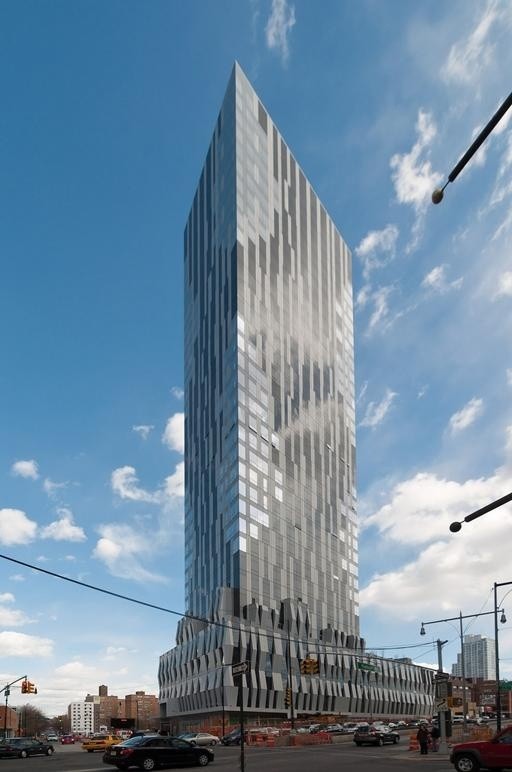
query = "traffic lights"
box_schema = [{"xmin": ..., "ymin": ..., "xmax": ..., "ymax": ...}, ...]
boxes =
[
  {"xmin": 285, "ymin": 687, "xmax": 291, "ymax": 706},
  {"xmin": 27, "ymin": 681, "xmax": 35, "ymax": 694},
  {"xmin": 21, "ymin": 681, "xmax": 27, "ymax": 693},
  {"xmin": 300, "ymin": 659, "xmax": 311, "ymax": 675},
  {"xmin": 310, "ymin": 658, "xmax": 319, "ymax": 675}
]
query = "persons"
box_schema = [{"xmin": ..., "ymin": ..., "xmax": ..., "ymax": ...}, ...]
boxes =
[
  {"xmin": 417, "ymin": 724, "xmax": 429, "ymax": 754},
  {"xmin": 430, "ymin": 723, "xmax": 440, "ymax": 752}
]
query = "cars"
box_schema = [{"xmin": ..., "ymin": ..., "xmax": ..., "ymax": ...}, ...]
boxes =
[
  {"xmin": 452, "ymin": 712, "xmax": 507, "ymax": 725},
  {"xmin": 0, "ymin": 738, "xmax": 54, "ymax": 759},
  {"xmin": 222, "ymin": 713, "xmax": 439, "ymax": 748},
  {"xmin": 449, "ymin": 725, "xmax": 512, "ymax": 771},
  {"xmin": 41, "ymin": 730, "xmax": 222, "ymax": 770}
]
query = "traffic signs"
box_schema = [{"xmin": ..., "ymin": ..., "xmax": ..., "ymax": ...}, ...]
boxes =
[
  {"xmin": 231, "ymin": 660, "xmax": 250, "ymax": 677},
  {"xmin": 354, "ymin": 661, "xmax": 381, "ymax": 671}
]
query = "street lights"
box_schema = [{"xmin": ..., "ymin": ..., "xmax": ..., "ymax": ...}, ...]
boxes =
[{"xmin": 419, "ymin": 607, "xmax": 508, "ymax": 742}]
